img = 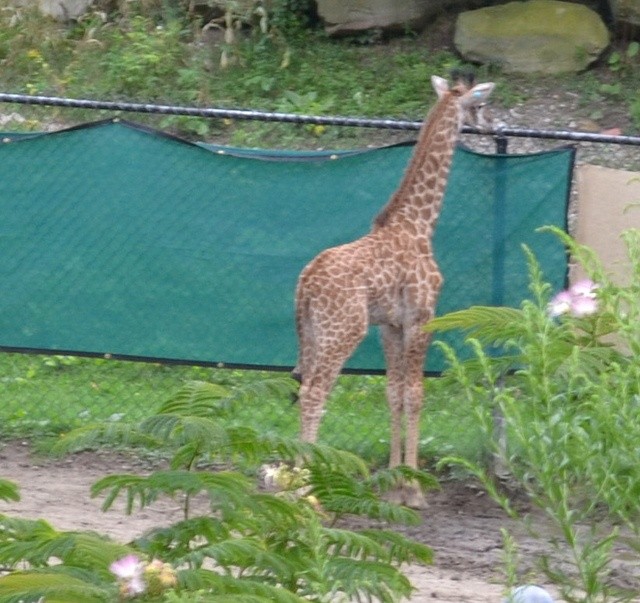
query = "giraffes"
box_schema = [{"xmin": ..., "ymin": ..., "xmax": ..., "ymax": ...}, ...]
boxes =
[{"xmin": 291, "ymin": 72, "xmax": 498, "ymax": 511}]
